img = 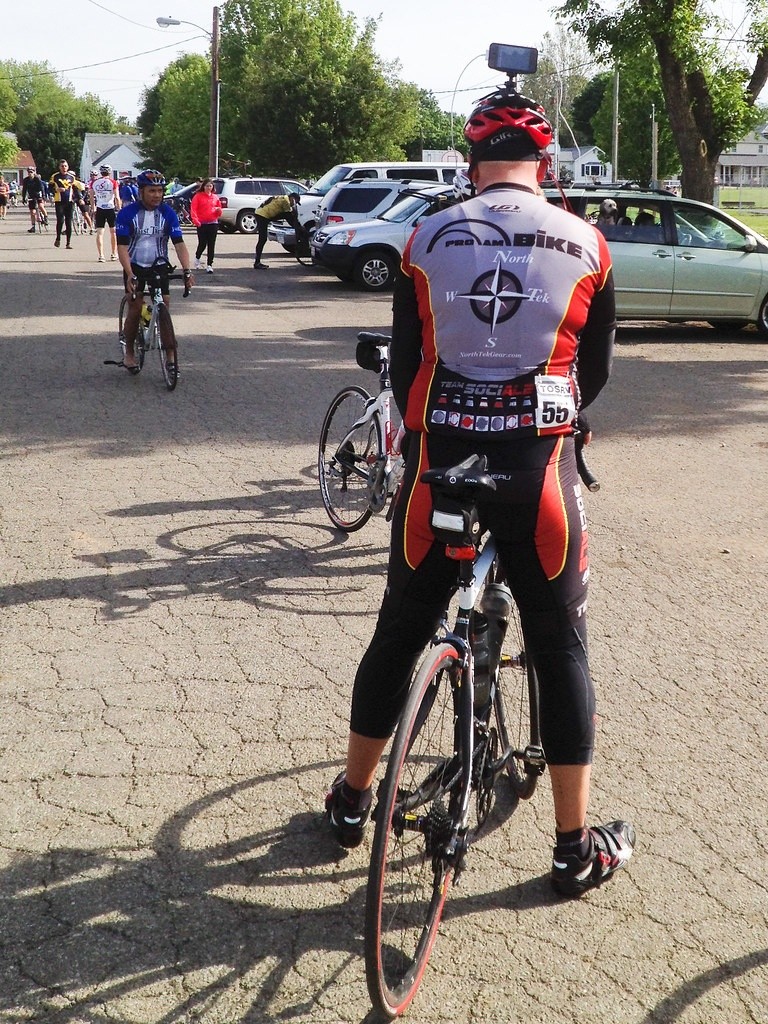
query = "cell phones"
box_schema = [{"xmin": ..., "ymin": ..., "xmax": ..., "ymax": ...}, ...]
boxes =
[{"xmin": 487, "ymin": 43, "xmax": 538, "ymax": 74}]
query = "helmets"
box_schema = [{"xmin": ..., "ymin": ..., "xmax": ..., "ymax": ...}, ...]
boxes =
[
  {"xmin": 459, "ymin": 86, "xmax": 553, "ymax": 160},
  {"xmin": 68, "ymin": 170, "xmax": 76, "ymax": 176},
  {"xmin": 27, "ymin": 166, "xmax": 36, "ymax": 172},
  {"xmin": 137, "ymin": 168, "xmax": 165, "ymax": 188},
  {"xmin": 89, "ymin": 170, "xmax": 98, "ymax": 176},
  {"xmin": 100, "ymin": 164, "xmax": 113, "ymax": 174}
]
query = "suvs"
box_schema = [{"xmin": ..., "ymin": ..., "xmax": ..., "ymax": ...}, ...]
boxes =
[
  {"xmin": 314, "ymin": 179, "xmax": 449, "ymax": 231},
  {"xmin": 540, "ymin": 181, "xmax": 768, "ymax": 337}
]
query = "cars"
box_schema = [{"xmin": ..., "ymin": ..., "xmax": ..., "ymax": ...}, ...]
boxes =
[
  {"xmin": 309, "ymin": 184, "xmax": 477, "ymax": 291},
  {"xmin": 163, "ymin": 181, "xmax": 201, "ymax": 222}
]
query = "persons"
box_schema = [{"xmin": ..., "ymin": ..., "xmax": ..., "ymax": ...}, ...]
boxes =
[
  {"xmin": 88, "ymin": 164, "xmax": 122, "ymax": 263},
  {"xmin": 119, "ymin": 176, "xmax": 139, "ymax": 207},
  {"xmin": 324, "ymin": 94, "xmax": 636, "ymax": 894},
  {"xmin": 117, "ymin": 169, "xmax": 195, "ymax": 378},
  {"xmin": 673, "ymin": 186, "xmax": 678, "ymax": 194},
  {"xmin": 165, "ymin": 178, "xmax": 183, "ymax": 196},
  {"xmin": 634, "ymin": 212, "xmax": 655, "ymax": 242},
  {"xmin": 190, "ymin": 178, "xmax": 223, "ymax": 273},
  {"xmin": 21, "ymin": 166, "xmax": 49, "ymax": 232},
  {"xmin": 8, "ymin": 180, "xmax": 18, "ymax": 207},
  {"xmin": 253, "ymin": 193, "xmax": 304, "ymax": 269},
  {"xmin": 0, "ymin": 175, "xmax": 9, "ymax": 220},
  {"xmin": 49, "ymin": 158, "xmax": 93, "ymax": 249}
]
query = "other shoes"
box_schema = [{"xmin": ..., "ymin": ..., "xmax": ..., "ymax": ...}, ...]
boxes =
[
  {"xmin": 110, "ymin": 254, "xmax": 120, "ymax": 262},
  {"xmin": 98, "ymin": 256, "xmax": 108, "ymax": 262},
  {"xmin": 28, "ymin": 228, "xmax": 35, "ymax": 234},
  {"xmin": 66, "ymin": 244, "xmax": 72, "ymax": 249},
  {"xmin": 54, "ymin": 238, "xmax": 60, "ymax": 248},
  {"xmin": 0, "ymin": 214, "xmax": 6, "ymax": 220},
  {"xmin": 165, "ymin": 360, "xmax": 181, "ymax": 379},
  {"xmin": 84, "ymin": 223, "xmax": 88, "ymax": 229},
  {"xmin": 44, "ymin": 216, "xmax": 49, "ymax": 225},
  {"xmin": 121, "ymin": 358, "xmax": 140, "ymax": 373},
  {"xmin": 63, "ymin": 231, "xmax": 67, "ymax": 235},
  {"xmin": 89, "ymin": 228, "xmax": 93, "ymax": 235},
  {"xmin": 254, "ymin": 262, "xmax": 269, "ymax": 270}
]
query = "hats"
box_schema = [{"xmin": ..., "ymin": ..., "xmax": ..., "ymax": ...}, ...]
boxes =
[{"xmin": 291, "ymin": 193, "xmax": 302, "ymax": 208}]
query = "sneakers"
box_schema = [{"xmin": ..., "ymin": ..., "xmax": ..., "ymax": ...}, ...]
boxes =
[
  {"xmin": 331, "ymin": 771, "xmax": 370, "ymax": 848},
  {"xmin": 206, "ymin": 264, "xmax": 212, "ymax": 274},
  {"xmin": 194, "ymin": 257, "xmax": 201, "ymax": 269},
  {"xmin": 550, "ymin": 820, "xmax": 635, "ymax": 899}
]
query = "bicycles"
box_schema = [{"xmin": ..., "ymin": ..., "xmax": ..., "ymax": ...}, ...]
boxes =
[
  {"xmin": 365, "ymin": 417, "xmax": 601, "ymax": 1018},
  {"xmin": 9, "ymin": 193, "xmax": 19, "ymax": 206},
  {"xmin": 72, "ymin": 190, "xmax": 90, "ymax": 235},
  {"xmin": 103, "ymin": 269, "xmax": 192, "ymax": 390},
  {"xmin": 163, "ymin": 197, "xmax": 193, "ymax": 227},
  {"xmin": 23, "ymin": 196, "xmax": 48, "ymax": 234},
  {"xmin": 318, "ymin": 332, "xmax": 406, "ymax": 533}
]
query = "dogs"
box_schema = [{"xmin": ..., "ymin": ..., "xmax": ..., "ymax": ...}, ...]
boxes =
[{"xmin": 597, "ymin": 198, "xmax": 618, "ymax": 225}]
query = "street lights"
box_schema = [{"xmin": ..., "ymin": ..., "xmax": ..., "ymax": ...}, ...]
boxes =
[{"xmin": 156, "ymin": 17, "xmax": 221, "ymax": 177}]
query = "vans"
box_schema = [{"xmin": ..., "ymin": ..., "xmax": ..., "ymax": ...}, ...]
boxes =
[
  {"xmin": 210, "ymin": 177, "xmax": 309, "ymax": 234},
  {"xmin": 266, "ymin": 162, "xmax": 470, "ymax": 257}
]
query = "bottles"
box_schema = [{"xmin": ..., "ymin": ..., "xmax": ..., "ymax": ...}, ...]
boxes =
[
  {"xmin": 393, "ymin": 419, "xmax": 406, "ymax": 451},
  {"xmin": 481, "ymin": 581, "xmax": 512, "ymax": 674},
  {"xmin": 474, "ymin": 610, "xmax": 491, "ymax": 708},
  {"xmin": 140, "ymin": 302, "xmax": 153, "ymax": 328}
]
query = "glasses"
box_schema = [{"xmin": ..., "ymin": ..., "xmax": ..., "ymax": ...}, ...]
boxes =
[{"xmin": 28, "ymin": 172, "xmax": 34, "ymax": 174}]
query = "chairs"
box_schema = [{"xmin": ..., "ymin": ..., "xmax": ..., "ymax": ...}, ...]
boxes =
[{"xmin": 617, "ymin": 217, "xmax": 633, "ymax": 241}]
